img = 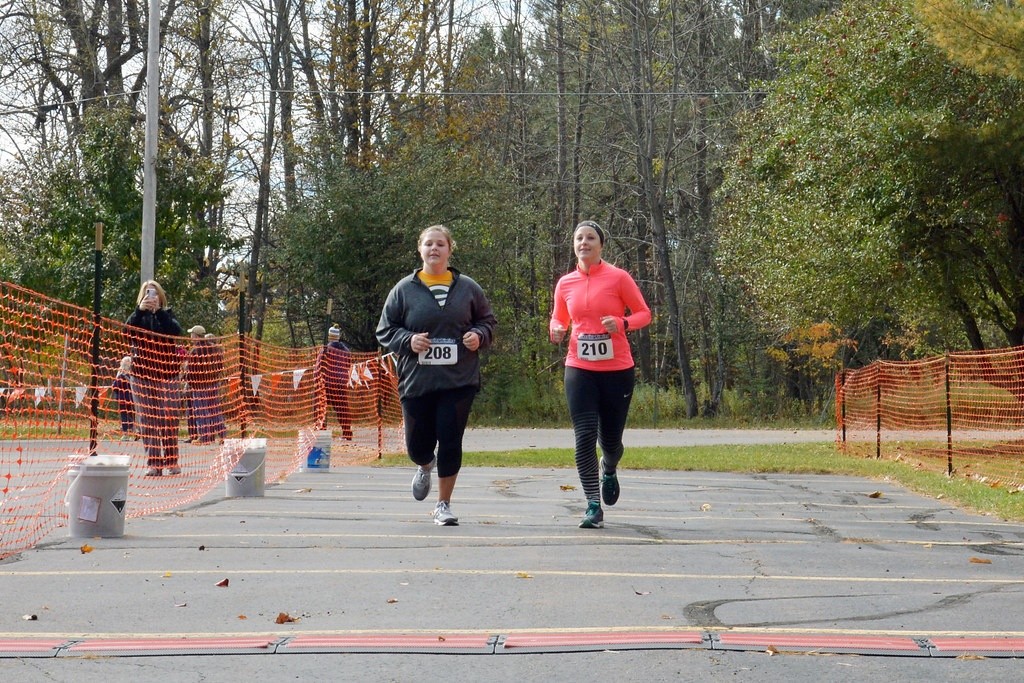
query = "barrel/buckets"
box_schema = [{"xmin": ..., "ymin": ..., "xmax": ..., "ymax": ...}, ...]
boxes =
[
  {"xmin": 220, "ymin": 438, "xmax": 266, "ymax": 498},
  {"xmin": 299, "ymin": 430, "xmax": 331, "ymax": 473},
  {"xmin": 66, "ymin": 454, "xmax": 130, "ymax": 538}
]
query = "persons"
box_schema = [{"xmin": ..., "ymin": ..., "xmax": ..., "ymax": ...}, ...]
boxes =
[
  {"xmin": 375, "ymin": 226, "xmax": 496, "ymax": 525},
  {"xmin": 313, "ymin": 324, "xmax": 354, "ymax": 442},
  {"xmin": 550, "ymin": 220, "xmax": 651, "ymax": 531},
  {"xmin": 111, "ymin": 280, "xmax": 226, "ymax": 476}
]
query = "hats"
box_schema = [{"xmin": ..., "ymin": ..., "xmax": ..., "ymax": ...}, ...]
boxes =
[
  {"xmin": 327, "ymin": 324, "xmax": 340, "ymax": 336},
  {"xmin": 204, "ymin": 332, "xmax": 215, "ymax": 339},
  {"xmin": 186, "ymin": 325, "xmax": 206, "ymax": 334}
]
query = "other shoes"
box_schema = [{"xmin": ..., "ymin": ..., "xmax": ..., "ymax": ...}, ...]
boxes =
[
  {"xmin": 120, "ymin": 435, "xmax": 130, "ymax": 440},
  {"xmin": 134, "ymin": 435, "xmax": 140, "ymax": 441},
  {"xmin": 191, "ymin": 438, "xmax": 211, "ymax": 445},
  {"xmin": 218, "ymin": 439, "xmax": 224, "ymax": 445},
  {"xmin": 144, "ymin": 467, "xmax": 163, "ymax": 476},
  {"xmin": 168, "ymin": 463, "xmax": 181, "ymax": 474},
  {"xmin": 183, "ymin": 435, "xmax": 198, "ymax": 442}
]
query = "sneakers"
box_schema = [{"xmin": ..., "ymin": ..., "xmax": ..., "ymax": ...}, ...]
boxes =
[
  {"xmin": 577, "ymin": 502, "xmax": 604, "ymax": 528},
  {"xmin": 599, "ymin": 456, "xmax": 620, "ymax": 506},
  {"xmin": 411, "ymin": 455, "xmax": 436, "ymax": 502},
  {"xmin": 431, "ymin": 500, "xmax": 460, "ymax": 526}
]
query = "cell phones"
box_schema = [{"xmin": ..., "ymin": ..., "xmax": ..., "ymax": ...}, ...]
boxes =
[{"xmin": 146, "ymin": 289, "xmax": 156, "ymax": 299}]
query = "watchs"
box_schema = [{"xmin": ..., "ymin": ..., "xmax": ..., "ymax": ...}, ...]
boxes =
[{"xmin": 621, "ymin": 317, "xmax": 630, "ymax": 331}]
